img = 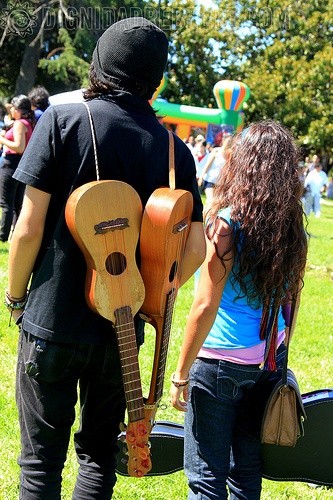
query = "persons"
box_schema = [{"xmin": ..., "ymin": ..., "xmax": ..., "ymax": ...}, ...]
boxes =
[
  {"xmin": 5, "ymin": 17, "xmax": 206, "ymax": 500},
  {"xmin": 184, "ymin": 134, "xmax": 210, "ymax": 162},
  {"xmin": 0, "ymin": 94, "xmax": 36, "ymax": 242},
  {"xmin": 28, "ymin": 87, "xmax": 50, "ymax": 122},
  {"xmin": 170, "ymin": 121, "xmax": 308, "ymax": 500},
  {"xmin": 198, "ymin": 132, "xmax": 234, "ymax": 226},
  {"xmin": 300, "ymin": 154, "xmax": 321, "ymax": 176},
  {"xmin": 304, "ymin": 163, "xmax": 327, "ymax": 218}
]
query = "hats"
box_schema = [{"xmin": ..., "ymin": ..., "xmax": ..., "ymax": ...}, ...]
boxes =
[{"xmin": 92, "ymin": 17, "xmax": 169, "ymax": 101}]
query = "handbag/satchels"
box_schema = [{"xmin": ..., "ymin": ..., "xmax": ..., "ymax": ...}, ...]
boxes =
[{"xmin": 260, "ymin": 368, "xmax": 308, "ymax": 448}]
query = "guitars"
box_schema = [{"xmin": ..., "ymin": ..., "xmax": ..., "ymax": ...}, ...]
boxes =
[
  {"xmin": 138, "ymin": 185, "xmax": 194, "ymax": 412},
  {"xmin": 67, "ymin": 176, "xmax": 153, "ymax": 480}
]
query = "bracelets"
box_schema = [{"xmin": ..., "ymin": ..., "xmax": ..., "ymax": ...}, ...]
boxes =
[
  {"xmin": 172, "ymin": 372, "xmax": 190, "ymax": 387},
  {"xmin": 5, "ymin": 291, "xmax": 28, "ymax": 327}
]
